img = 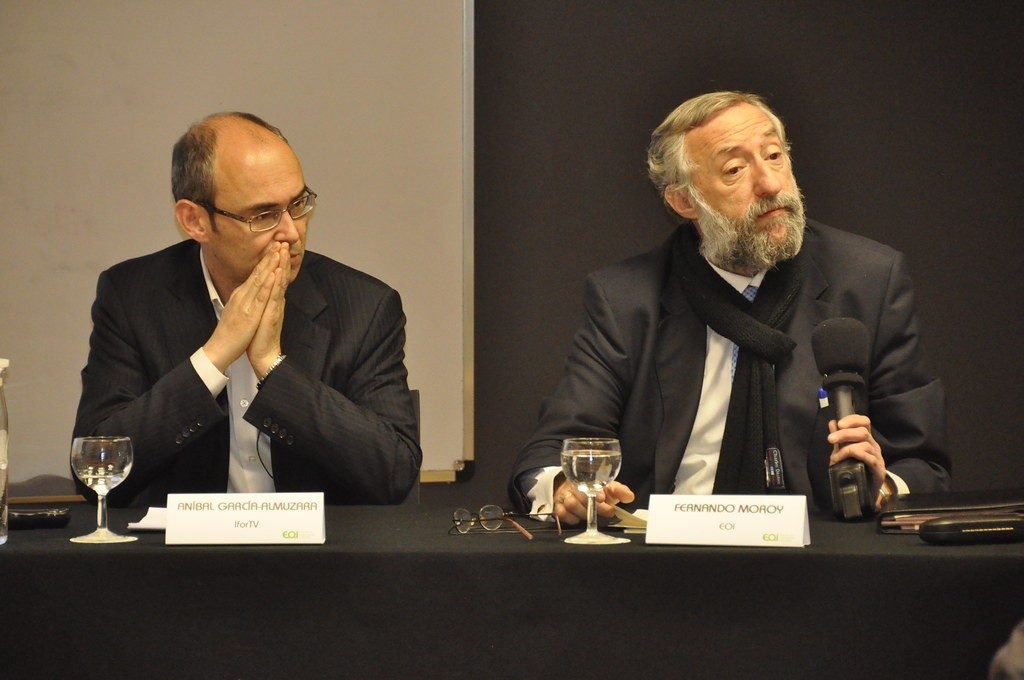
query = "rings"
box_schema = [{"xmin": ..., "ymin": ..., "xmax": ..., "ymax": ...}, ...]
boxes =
[{"xmin": 561, "ymin": 492, "xmax": 572, "ymax": 499}]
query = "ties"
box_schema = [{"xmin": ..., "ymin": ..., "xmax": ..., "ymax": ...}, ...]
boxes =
[{"xmin": 732, "ymin": 285, "xmax": 758, "ymax": 382}]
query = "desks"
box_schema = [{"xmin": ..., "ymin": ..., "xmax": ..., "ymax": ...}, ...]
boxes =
[{"xmin": 0, "ymin": 504, "xmax": 1024, "ymax": 680}]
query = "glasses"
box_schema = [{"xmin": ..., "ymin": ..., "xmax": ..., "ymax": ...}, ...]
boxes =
[
  {"xmin": 448, "ymin": 505, "xmax": 562, "ymax": 540},
  {"xmin": 194, "ymin": 186, "xmax": 317, "ymax": 232}
]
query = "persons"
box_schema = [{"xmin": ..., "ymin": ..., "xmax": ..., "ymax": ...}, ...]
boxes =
[
  {"xmin": 511, "ymin": 92, "xmax": 954, "ymax": 526},
  {"xmin": 71, "ymin": 111, "xmax": 424, "ymax": 506}
]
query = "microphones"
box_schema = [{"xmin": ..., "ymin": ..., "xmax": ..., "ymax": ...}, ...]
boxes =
[{"xmin": 811, "ymin": 318, "xmax": 878, "ymax": 520}]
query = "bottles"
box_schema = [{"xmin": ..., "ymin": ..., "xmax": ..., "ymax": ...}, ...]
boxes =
[{"xmin": 0, "ymin": 370, "xmax": 12, "ymax": 543}]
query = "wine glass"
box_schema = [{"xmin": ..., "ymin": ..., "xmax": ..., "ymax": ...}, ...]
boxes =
[
  {"xmin": 70, "ymin": 435, "xmax": 134, "ymax": 543},
  {"xmin": 560, "ymin": 436, "xmax": 622, "ymax": 545}
]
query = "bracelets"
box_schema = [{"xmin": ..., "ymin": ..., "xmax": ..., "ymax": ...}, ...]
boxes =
[
  {"xmin": 878, "ymin": 483, "xmax": 892, "ymax": 497},
  {"xmin": 256, "ymin": 353, "xmax": 286, "ymax": 391}
]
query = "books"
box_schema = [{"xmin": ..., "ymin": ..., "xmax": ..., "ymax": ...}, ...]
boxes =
[{"xmin": 876, "ymin": 491, "xmax": 1024, "ymax": 535}]
